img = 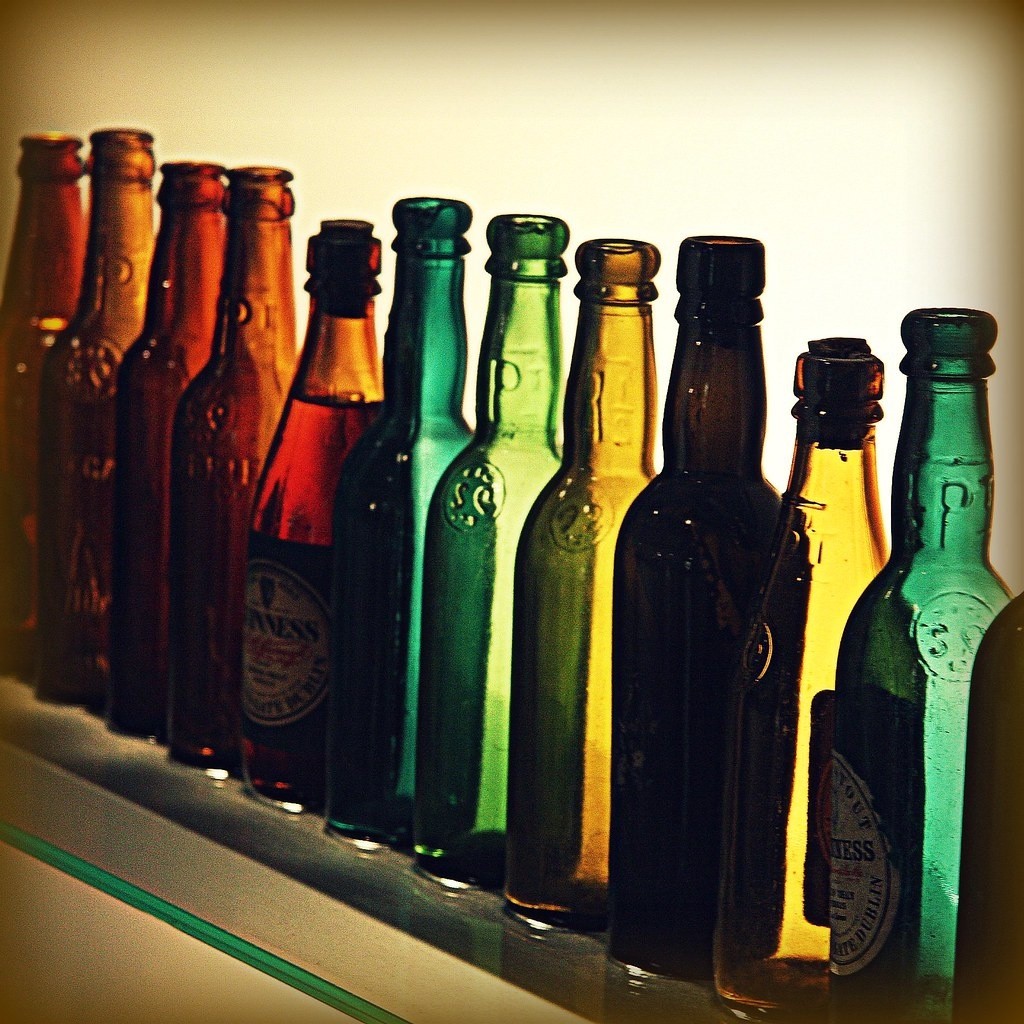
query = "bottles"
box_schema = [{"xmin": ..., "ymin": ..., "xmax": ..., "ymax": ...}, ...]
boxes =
[
  {"xmin": 0, "ymin": 136, "xmax": 84, "ymax": 677},
  {"xmin": 713, "ymin": 338, "xmax": 887, "ymax": 1024},
  {"xmin": 507, "ymin": 238, "xmax": 659, "ymax": 931},
  {"xmin": 322, "ymin": 198, "xmax": 476, "ymax": 849},
  {"xmin": 110, "ymin": 162, "xmax": 226, "ymax": 740},
  {"xmin": 829, "ymin": 307, "xmax": 1013, "ymax": 1024},
  {"xmin": 237, "ymin": 219, "xmax": 385, "ymax": 813},
  {"xmin": 609, "ymin": 236, "xmax": 780, "ymax": 978},
  {"xmin": 34, "ymin": 130, "xmax": 155, "ymax": 706},
  {"xmin": 415, "ymin": 215, "xmax": 569, "ymax": 890},
  {"xmin": 168, "ymin": 168, "xmax": 299, "ymax": 780},
  {"xmin": 949, "ymin": 591, "xmax": 1024, "ymax": 1024}
]
